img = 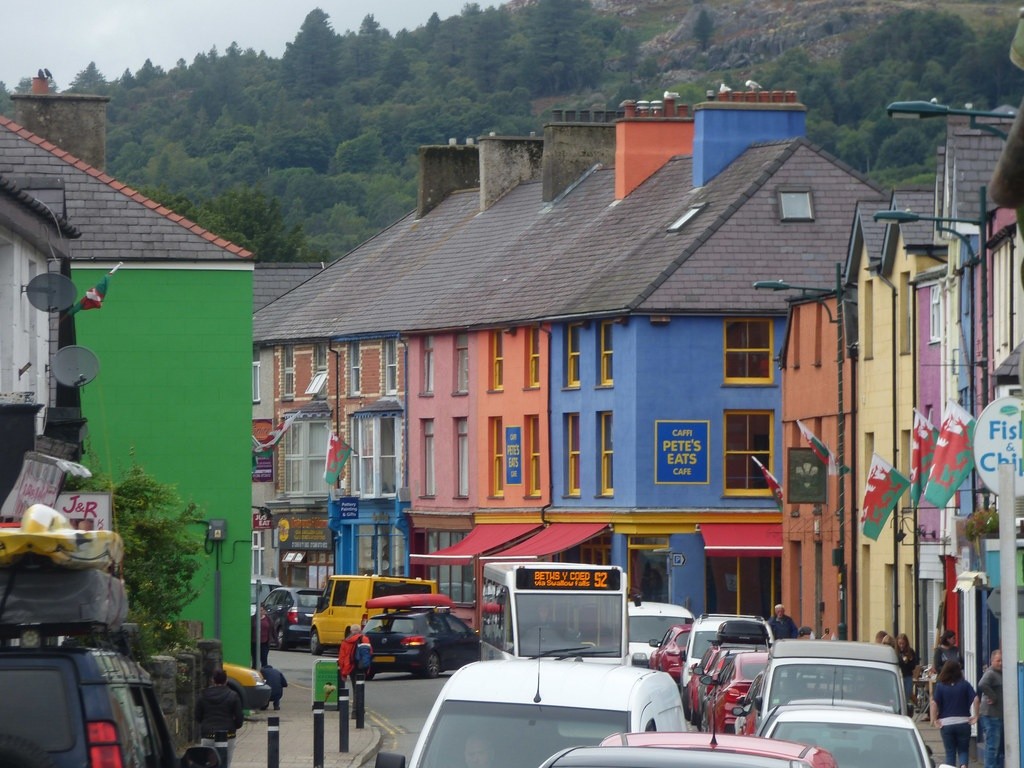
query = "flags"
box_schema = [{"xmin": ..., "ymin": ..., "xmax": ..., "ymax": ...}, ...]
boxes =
[
  {"xmin": 761, "ymin": 464, "xmax": 783, "ymax": 516},
  {"xmin": 909, "ymin": 413, "xmax": 940, "ymax": 510},
  {"xmin": 860, "ymin": 451, "xmax": 911, "ymax": 542},
  {"xmin": 322, "ymin": 430, "xmax": 352, "ymax": 484},
  {"xmin": 256, "ymin": 410, "xmax": 302, "ymax": 458},
  {"xmin": 923, "ymin": 394, "xmax": 978, "ymax": 511},
  {"xmin": 797, "ymin": 421, "xmax": 850, "ymax": 476}
]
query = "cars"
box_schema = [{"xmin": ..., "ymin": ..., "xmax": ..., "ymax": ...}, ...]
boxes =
[
  {"xmin": 222, "ymin": 660, "xmax": 273, "ymax": 721},
  {"xmin": 362, "ymin": 592, "xmax": 481, "ymax": 679},
  {"xmin": 260, "ymin": 586, "xmax": 326, "ymax": 652},
  {"xmin": 647, "ymin": 623, "xmax": 694, "ymax": 689},
  {"xmin": 536, "ymin": 619, "xmax": 936, "ymax": 768}
]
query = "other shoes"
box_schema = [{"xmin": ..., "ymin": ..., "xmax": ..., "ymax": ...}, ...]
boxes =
[{"xmin": 274, "ymin": 706, "xmax": 280, "ymax": 710}]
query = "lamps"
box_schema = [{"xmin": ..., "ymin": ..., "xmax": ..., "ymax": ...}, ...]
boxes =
[
  {"xmin": 251, "ymin": 505, "xmax": 273, "ymax": 519},
  {"xmin": 897, "ymin": 517, "xmax": 937, "ymax": 543}
]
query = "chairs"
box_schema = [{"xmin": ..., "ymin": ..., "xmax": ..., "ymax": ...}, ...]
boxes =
[{"xmin": 872, "ymin": 735, "xmax": 899, "ymax": 758}]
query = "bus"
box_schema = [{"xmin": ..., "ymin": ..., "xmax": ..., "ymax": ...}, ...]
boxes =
[
  {"xmin": 479, "ymin": 560, "xmax": 643, "ymax": 665},
  {"xmin": 308, "ymin": 574, "xmax": 439, "ymax": 656}
]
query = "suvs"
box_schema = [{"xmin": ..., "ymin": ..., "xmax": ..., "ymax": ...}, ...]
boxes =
[{"xmin": 0, "ymin": 504, "xmax": 224, "ymax": 768}]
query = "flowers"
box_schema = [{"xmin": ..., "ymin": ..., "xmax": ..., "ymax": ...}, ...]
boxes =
[{"xmin": 965, "ymin": 507, "xmax": 999, "ymax": 542}]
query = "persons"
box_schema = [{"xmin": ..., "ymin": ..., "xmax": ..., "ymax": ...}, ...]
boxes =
[
  {"xmin": 930, "ymin": 660, "xmax": 980, "ymax": 768},
  {"xmin": 339, "ymin": 625, "xmax": 373, "ymax": 719},
  {"xmin": 464, "ymin": 732, "xmax": 497, "ymax": 768},
  {"xmin": 799, "ymin": 626, "xmax": 813, "ymax": 639},
  {"xmin": 260, "ymin": 665, "xmax": 288, "ymax": 710},
  {"xmin": 876, "ymin": 631, "xmax": 922, "ymax": 705},
  {"xmin": 251, "ymin": 603, "xmax": 279, "ymax": 670},
  {"xmin": 768, "ymin": 604, "xmax": 798, "ymax": 640},
  {"xmin": 922, "ymin": 631, "xmax": 964, "ymax": 723},
  {"xmin": 194, "ymin": 669, "xmax": 244, "ymax": 768},
  {"xmin": 978, "ymin": 649, "xmax": 1005, "ymax": 767}
]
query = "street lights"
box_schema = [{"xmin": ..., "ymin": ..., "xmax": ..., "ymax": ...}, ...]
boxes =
[
  {"xmin": 753, "ymin": 262, "xmax": 838, "ymax": 642},
  {"xmin": 873, "ymin": 185, "xmax": 992, "ymax": 516}
]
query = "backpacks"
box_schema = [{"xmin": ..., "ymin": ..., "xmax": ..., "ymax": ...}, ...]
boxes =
[{"xmin": 354, "ymin": 636, "xmax": 371, "ymax": 671}]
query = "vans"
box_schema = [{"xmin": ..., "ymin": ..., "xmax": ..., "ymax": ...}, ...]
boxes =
[
  {"xmin": 405, "ymin": 657, "xmax": 688, "ymax": 768},
  {"xmin": 681, "ymin": 613, "xmax": 777, "ymax": 721},
  {"xmin": 626, "ymin": 601, "xmax": 697, "ymax": 668}
]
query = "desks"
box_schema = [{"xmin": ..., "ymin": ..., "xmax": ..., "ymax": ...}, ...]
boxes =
[{"xmin": 912, "ymin": 679, "xmax": 930, "ymax": 724}]
